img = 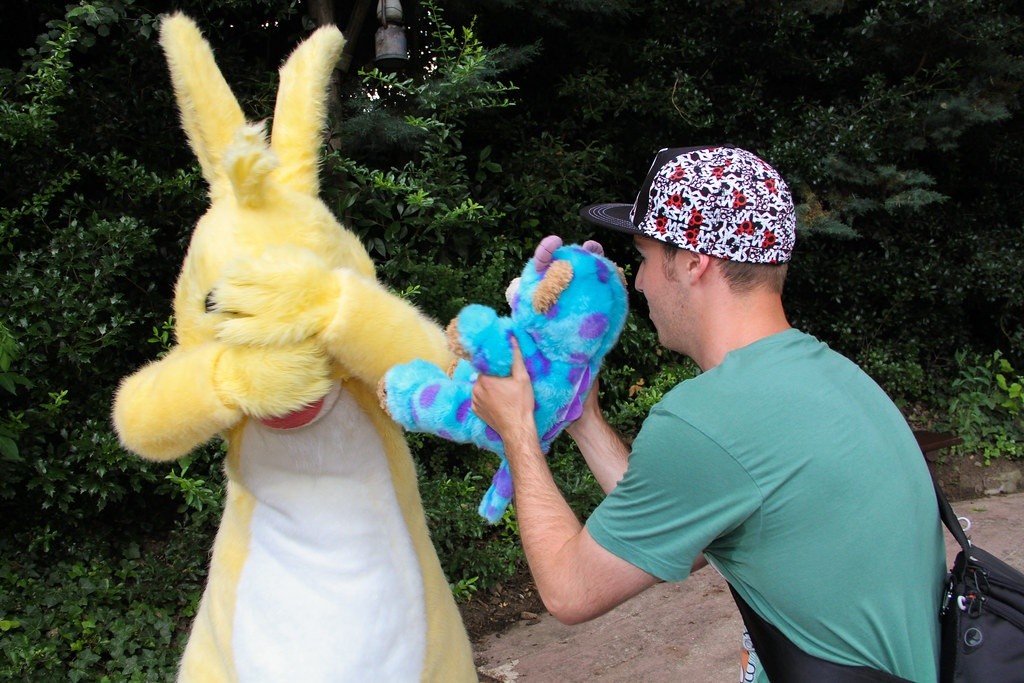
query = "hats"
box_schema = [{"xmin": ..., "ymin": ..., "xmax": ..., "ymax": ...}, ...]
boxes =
[{"xmin": 580, "ymin": 143, "xmax": 797, "ymax": 264}]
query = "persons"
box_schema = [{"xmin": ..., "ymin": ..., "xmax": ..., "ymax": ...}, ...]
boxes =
[{"xmin": 470, "ymin": 143, "xmax": 948, "ymax": 683}]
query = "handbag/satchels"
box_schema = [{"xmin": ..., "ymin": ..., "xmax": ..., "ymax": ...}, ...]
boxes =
[{"xmin": 940, "ymin": 543, "xmax": 1024, "ymax": 683}]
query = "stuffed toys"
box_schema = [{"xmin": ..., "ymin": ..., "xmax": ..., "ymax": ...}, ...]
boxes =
[
  {"xmin": 376, "ymin": 234, "xmax": 629, "ymax": 528},
  {"xmin": 113, "ymin": 10, "xmax": 479, "ymax": 683}
]
